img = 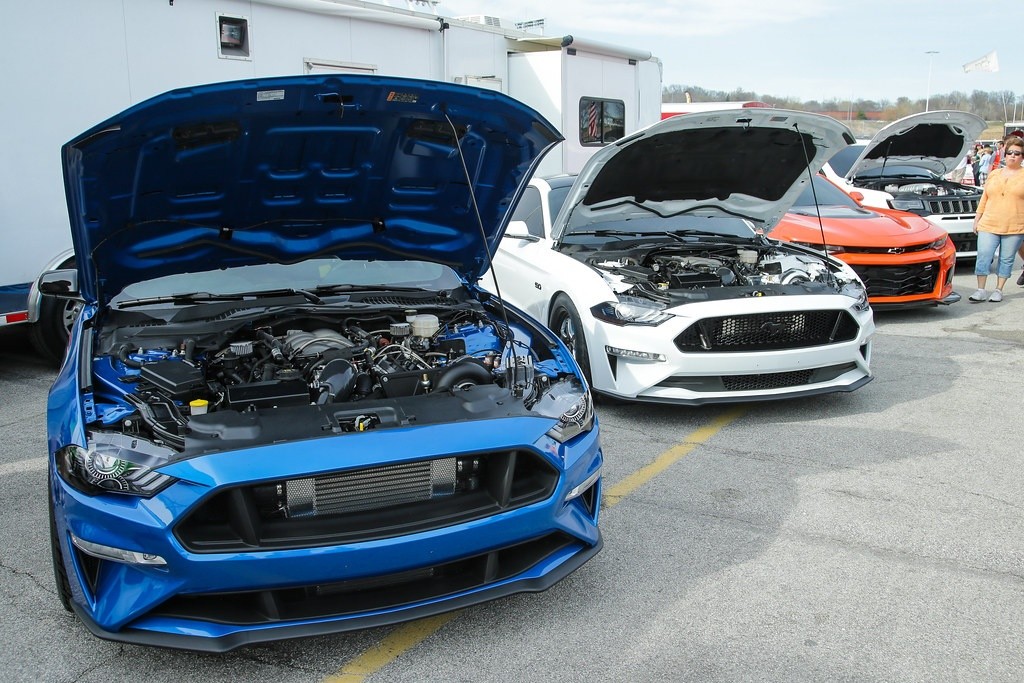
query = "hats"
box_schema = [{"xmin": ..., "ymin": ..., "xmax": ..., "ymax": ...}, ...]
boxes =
[
  {"xmin": 1011, "ymin": 130, "xmax": 1023, "ymax": 138},
  {"xmin": 982, "ymin": 147, "xmax": 992, "ymax": 151},
  {"xmin": 984, "ymin": 144, "xmax": 990, "ymax": 147}
]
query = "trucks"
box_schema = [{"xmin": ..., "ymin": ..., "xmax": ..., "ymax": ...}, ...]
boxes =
[{"xmin": 1, "ymin": 0, "xmax": 661, "ymax": 357}]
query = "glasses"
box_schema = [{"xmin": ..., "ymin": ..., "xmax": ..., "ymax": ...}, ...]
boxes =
[{"xmin": 1006, "ymin": 151, "xmax": 1020, "ymax": 155}]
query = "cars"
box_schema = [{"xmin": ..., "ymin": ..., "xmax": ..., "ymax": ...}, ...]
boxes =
[
  {"xmin": 34, "ymin": 71, "xmax": 621, "ymax": 636},
  {"xmin": 826, "ymin": 106, "xmax": 994, "ymax": 256},
  {"xmin": 762, "ymin": 167, "xmax": 965, "ymax": 300},
  {"xmin": 497, "ymin": 110, "xmax": 894, "ymax": 371}
]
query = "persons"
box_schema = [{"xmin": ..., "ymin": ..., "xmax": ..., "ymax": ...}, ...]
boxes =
[
  {"xmin": 1016, "ymin": 240, "xmax": 1024, "ymax": 287},
  {"xmin": 968, "ymin": 139, "xmax": 1024, "ymax": 303},
  {"xmin": 951, "ymin": 130, "xmax": 1024, "ymax": 188}
]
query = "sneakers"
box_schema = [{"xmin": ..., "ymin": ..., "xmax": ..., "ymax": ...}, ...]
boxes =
[
  {"xmin": 989, "ymin": 289, "xmax": 1003, "ymax": 301},
  {"xmin": 969, "ymin": 289, "xmax": 987, "ymax": 301}
]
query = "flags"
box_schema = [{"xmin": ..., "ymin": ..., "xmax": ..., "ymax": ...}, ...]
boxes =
[{"xmin": 589, "ymin": 101, "xmax": 597, "ymax": 139}]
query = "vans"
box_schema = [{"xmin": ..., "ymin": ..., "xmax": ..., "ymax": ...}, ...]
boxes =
[{"xmin": 661, "ymin": 101, "xmax": 772, "ymax": 124}]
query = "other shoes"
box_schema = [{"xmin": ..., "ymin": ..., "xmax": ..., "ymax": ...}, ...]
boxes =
[{"xmin": 1016, "ymin": 271, "xmax": 1024, "ymax": 285}]
